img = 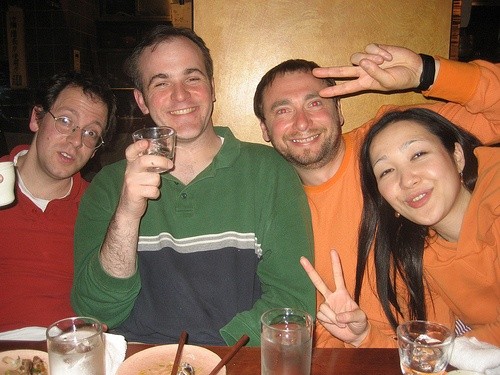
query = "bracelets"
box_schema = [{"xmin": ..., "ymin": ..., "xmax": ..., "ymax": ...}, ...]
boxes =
[{"xmin": 415, "ymin": 53, "xmax": 436, "ymax": 93}]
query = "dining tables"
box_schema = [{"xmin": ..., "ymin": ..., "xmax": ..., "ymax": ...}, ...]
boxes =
[{"xmin": 0, "ymin": 340, "xmax": 459, "ymax": 375}]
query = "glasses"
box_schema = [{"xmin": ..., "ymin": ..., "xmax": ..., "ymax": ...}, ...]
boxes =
[{"xmin": 47, "ymin": 108, "xmax": 104, "ymax": 149}]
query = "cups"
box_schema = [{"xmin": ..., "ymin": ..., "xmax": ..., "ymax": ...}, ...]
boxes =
[
  {"xmin": 260, "ymin": 307, "xmax": 313, "ymax": 375},
  {"xmin": 46, "ymin": 317, "xmax": 105, "ymax": 375},
  {"xmin": 397, "ymin": 320, "xmax": 454, "ymax": 375},
  {"xmin": 0, "ymin": 161, "xmax": 16, "ymax": 207},
  {"xmin": 134, "ymin": 127, "xmax": 176, "ymax": 173}
]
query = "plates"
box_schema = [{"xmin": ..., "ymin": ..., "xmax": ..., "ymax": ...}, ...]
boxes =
[
  {"xmin": 117, "ymin": 344, "xmax": 227, "ymax": 375},
  {"xmin": 1, "ymin": 349, "xmax": 50, "ymax": 375}
]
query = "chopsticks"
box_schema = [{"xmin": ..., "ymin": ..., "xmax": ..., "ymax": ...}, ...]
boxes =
[{"xmin": 171, "ymin": 331, "xmax": 250, "ymax": 375}]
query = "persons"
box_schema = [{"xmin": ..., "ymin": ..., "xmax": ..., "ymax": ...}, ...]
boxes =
[
  {"xmin": 72, "ymin": 28, "xmax": 316, "ymax": 349},
  {"xmin": 0, "ymin": 71, "xmax": 118, "ymax": 340},
  {"xmin": 254, "ymin": 44, "xmax": 500, "ymax": 346},
  {"xmin": 299, "ymin": 107, "xmax": 500, "ymax": 351}
]
